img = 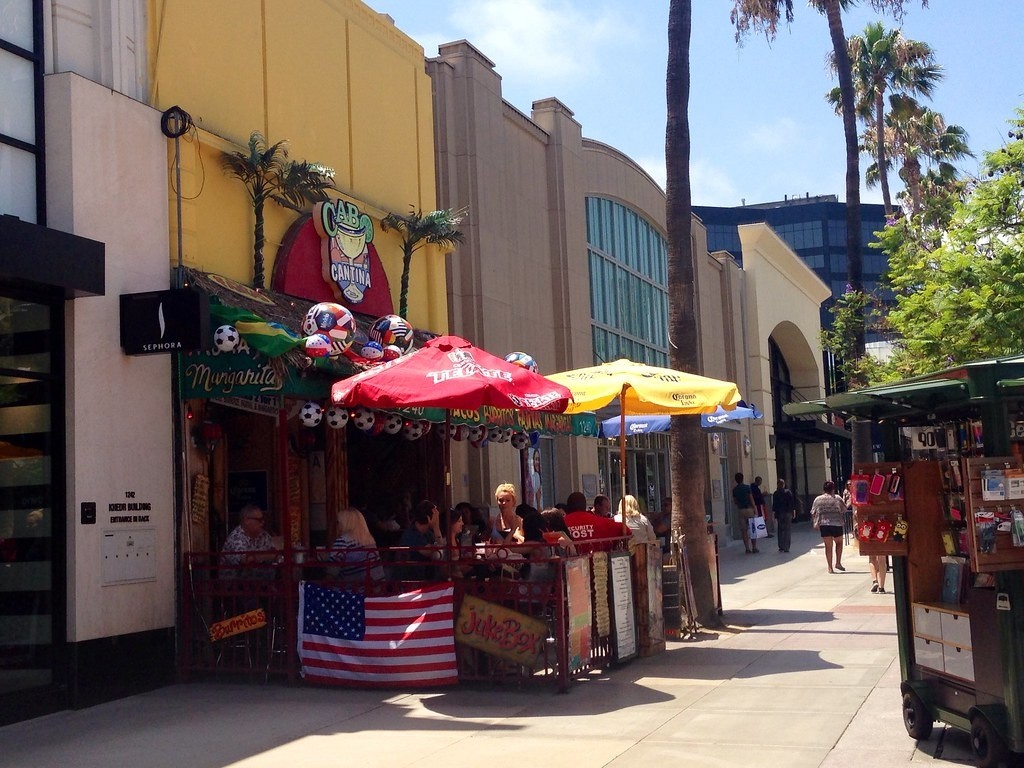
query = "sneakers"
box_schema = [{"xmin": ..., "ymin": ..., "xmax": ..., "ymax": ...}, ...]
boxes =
[
  {"xmin": 878, "ymin": 587, "xmax": 885, "ymax": 594},
  {"xmin": 871, "ymin": 580, "xmax": 879, "ymax": 592}
]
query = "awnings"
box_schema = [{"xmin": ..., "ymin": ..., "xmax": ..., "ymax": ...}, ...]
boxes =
[{"xmin": 173, "ymin": 263, "xmax": 600, "ymax": 438}]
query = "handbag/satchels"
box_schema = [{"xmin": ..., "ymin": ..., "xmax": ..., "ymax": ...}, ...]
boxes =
[{"xmin": 748, "ymin": 516, "xmax": 768, "ymax": 539}]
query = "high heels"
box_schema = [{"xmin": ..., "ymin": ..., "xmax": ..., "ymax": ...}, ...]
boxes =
[{"xmin": 784, "ymin": 549, "xmax": 789, "ymax": 552}]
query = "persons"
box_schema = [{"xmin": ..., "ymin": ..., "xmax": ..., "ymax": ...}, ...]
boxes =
[
  {"xmin": 374, "ymin": 484, "xmax": 671, "ymax": 582},
  {"xmin": 810, "ymin": 480, "xmax": 889, "ymax": 594},
  {"xmin": 732, "ymin": 473, "xmax": 796, "ymax": 552},
  {"xmin": 28, "ymin": 511, "xmax": 43, "ymax": 528},
  {"xmin": 219, "ymin": 505, "xmax": 289, "ymax": 613},
  {"xmin": 525, "ymin": 449, "xmax": 543, "ymax": 513},
  {"xmin": 316, "ymin": 508, "xmax": 385, "ymax": 587}
]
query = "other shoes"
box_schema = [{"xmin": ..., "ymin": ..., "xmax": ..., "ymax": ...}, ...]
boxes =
[
  {"xmin": 779, "ymin": 547, "xmax": 784, "ymax": 552},
  {"xmin": 835, "ymin": 565, "xmax": 845, "ymax": 571},
  {"xmin": 745, "ymin": 550, "xmax": 751, "ymax": 554},
  {"xmin": 752, "ymin": 549, "xmax": 759, "ymax": 554},
  {"xmin": 828, "ymin": 569, "xmax": 834, "ymax": 574}
]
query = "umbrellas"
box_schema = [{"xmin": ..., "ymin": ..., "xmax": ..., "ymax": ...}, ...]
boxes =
[
  {"xmin": 601, "ymin": 401, "xmax": 763, "ymax": 439},
  {"xmin": 541, "ymin": 360, "xmax": 744, "ymax": 536},
  {"xmin": 330, "ymin": 336, "xmax": 577, "ymax": 539}
]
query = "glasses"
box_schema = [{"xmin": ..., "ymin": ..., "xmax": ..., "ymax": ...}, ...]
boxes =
[{"xmin": 246, "ymin": 516, "xmax": 266, "ymax": 522}]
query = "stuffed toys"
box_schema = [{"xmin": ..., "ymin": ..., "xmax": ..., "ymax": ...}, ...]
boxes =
[
  {"xmin": 873, "ymin": 520, "xmax": 892, "ymax": 543},
  {"xmin": 860, "ymin": 522, "xmax": 874, "ymax": 542}
]
